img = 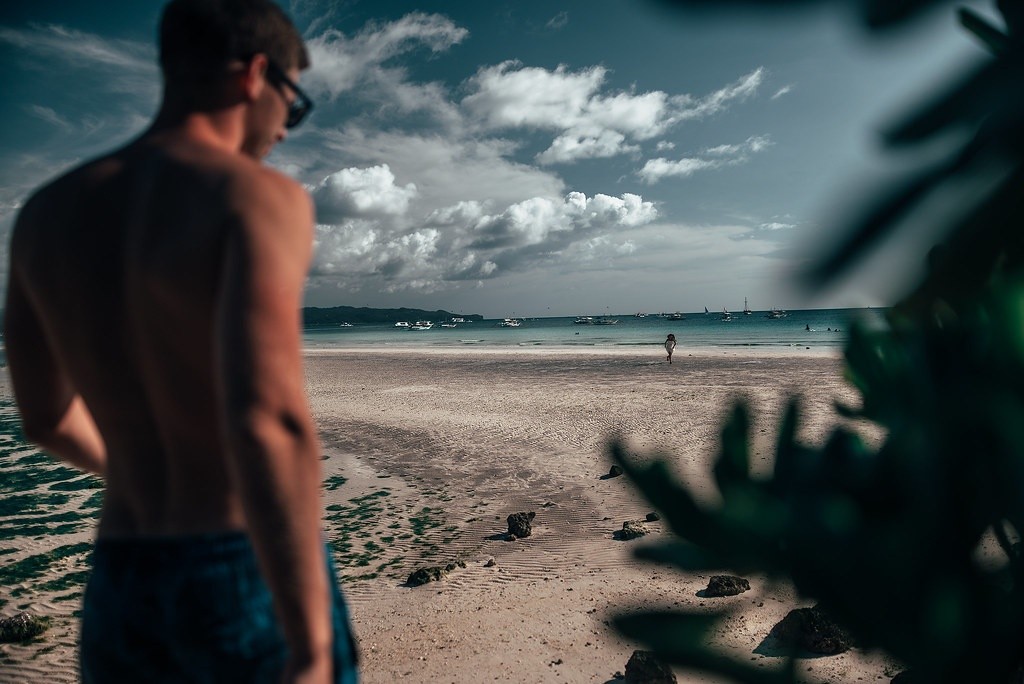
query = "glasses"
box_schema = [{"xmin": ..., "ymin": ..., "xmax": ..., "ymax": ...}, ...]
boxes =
[{"xmin": 270, "ymin": 62, "xmax": 314, "ymax": 128}]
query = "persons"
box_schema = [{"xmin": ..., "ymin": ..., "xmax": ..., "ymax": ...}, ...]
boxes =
[
  {"xmin": 3, "ymin": 0, "xmax": 368, "ymax": 683},
  {"xmin": 665, "ymin": 333, "xmax": 677, "ymax": 364}
]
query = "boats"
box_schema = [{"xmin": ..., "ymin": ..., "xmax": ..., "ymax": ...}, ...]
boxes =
[
  {"xmin": 395, "ymin": 318, "xmax": 433, "ymax": 331},
  {"xmin": 499, "ymin": 318, "xmax": 521, "ymax": 327},
  {"xmin": 340, "ymin": 322, "xmax": 353, "ymax": 327},
  {"xmin": 594, "ymin": 320, "xmax": 619, "ymax": 325},
  {"xmin": 719, "ymin": 308, "xmax": 740, "ymax": 322},
  {"xmin": 635, "ymin": 312, "xmax": 648, "ymax": 317},
  {"xmin": 442, "ymin": 324, "xmax": 458, "ymax": 328},
  {"xmin": 451, "ymin": 317, "xmax": 472, "ymax": 323},
  {"xmin": 656, "ymin": 312, "xmax": 687, "ymax": 319},
  {"xmin": 767, "ymin": 306, "xmax": 793, "ymax": 318},
  {"xmin": 574, "ymin": 316, "xmax": 593, "ymax": 324}
]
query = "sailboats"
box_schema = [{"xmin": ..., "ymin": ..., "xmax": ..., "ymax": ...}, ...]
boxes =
[{"xmin": 744, "ymin": 297, "xmax": 752, "ymax": 314}]
